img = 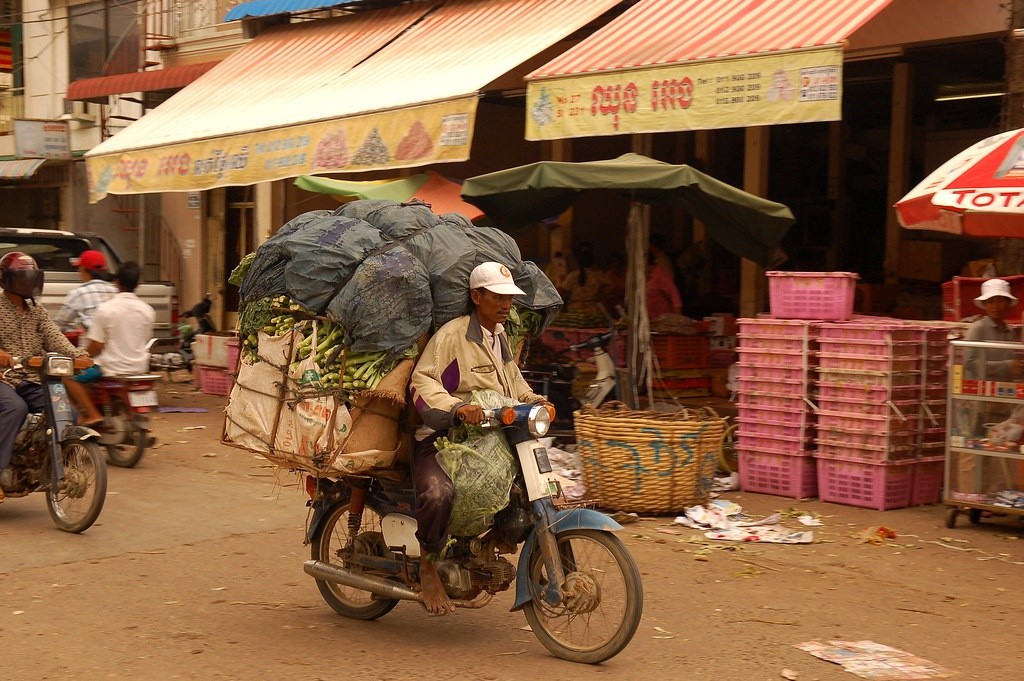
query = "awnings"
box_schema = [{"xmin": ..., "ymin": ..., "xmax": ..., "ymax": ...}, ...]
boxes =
[
  {"xmin": 84, "ymin": 0, "xmax": 620, "ymax": 203},
  {"xmin": 521, "ymin": 0, "xmax": 896, "ymax": 143}
]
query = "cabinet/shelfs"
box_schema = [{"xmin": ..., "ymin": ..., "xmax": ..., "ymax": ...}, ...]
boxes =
[{"xmin": 941, "ymin": 340, "xmax": 1024, "ymax": 531}]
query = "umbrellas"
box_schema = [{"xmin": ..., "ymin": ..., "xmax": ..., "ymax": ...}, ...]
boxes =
[
  {"xmin": 461, "ymin": 153, "xmax": 795, "ymax": 411},
  {"xmin": 293, "ymin": 168, "xmax": 486, "ymax": 221},
  {"xmin": 893, "ymin": 124, "xmax": 1024, "ymax": 237}
]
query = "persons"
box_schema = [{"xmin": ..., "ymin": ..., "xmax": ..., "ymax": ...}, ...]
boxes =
[
  {"xmin": 959, "ymin": 278, "xmax": 1019, "ymax": 422},
  {"xmin": 53, "ymin": 252, "xmax": 157, "ymax": 427},
  {"xmin": 550, "ymin": 231, "xmax": 708, "ymax": 320},
  {"xmin": 410, "ymin": 262, "xmax": 547, "ymax": 614},
  {"xmin": 0, "ymin": 250, "xmax": 93, "ymax": 474}
]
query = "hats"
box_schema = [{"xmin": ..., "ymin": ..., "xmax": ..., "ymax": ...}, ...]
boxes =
[
  {"xmin": 469, "ymin": 261, "xmax": 526, "ymax": 295},
  {"xmin": 73, "ymin": 250, "xmax": 106, "ymax": 271},
  {"xmin": 974, "ymin": 279, "xmax": 1019, "ymax": 310}
]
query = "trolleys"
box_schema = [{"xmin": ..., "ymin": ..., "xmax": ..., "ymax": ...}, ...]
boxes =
[{"xmin": 940, "ymin": 340, "xmax": 1024, "ymax": 531}]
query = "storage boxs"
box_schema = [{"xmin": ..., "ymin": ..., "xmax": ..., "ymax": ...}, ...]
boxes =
[
  {"xmin": 731, "ymin": 271, "xmax": 1023, "ymax": 512},
  {"xmin": 529, "ymin": 311, "xmax": 738, "ymax": 398},
  {"xmin": 189, "ymin": 334, "xmax": 241, "ymax": 395}
]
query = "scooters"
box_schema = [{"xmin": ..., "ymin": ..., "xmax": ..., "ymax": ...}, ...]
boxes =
[{"xmin": 516, "ymin": 323, "xmax": 630, "ymax": 443}]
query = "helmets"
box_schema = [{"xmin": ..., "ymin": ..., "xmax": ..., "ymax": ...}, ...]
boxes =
[{"xmin": 0, "ymin": 251, "xmax": 40, "ymax": 292}]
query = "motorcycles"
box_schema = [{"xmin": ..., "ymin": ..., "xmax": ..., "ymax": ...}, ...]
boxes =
[
  {"xmin": 302, "ymin": 394, "xmax": 646, "ymax": 666},
  {"xmin": 0, "ymin": 349, "xmax": 109, "ymax": 534},
  {"xmin": 60, "ymin": 322, "xmax": 163, "ymax": 469}
]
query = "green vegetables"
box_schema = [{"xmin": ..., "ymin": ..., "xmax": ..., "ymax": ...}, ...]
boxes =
[{"xmin": 228, "ymin": 250, "xmax": 553, "ymax": 555}]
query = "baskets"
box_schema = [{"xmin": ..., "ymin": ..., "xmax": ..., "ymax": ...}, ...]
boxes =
[{"xmin": 572, "ymin": 400, "xmax": 726, "ymax": 516}]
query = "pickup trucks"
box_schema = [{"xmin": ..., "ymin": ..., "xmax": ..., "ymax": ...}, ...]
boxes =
[{"xmin": 0, "ymin": 226, "xmax": 182, "ymax": 355}]
